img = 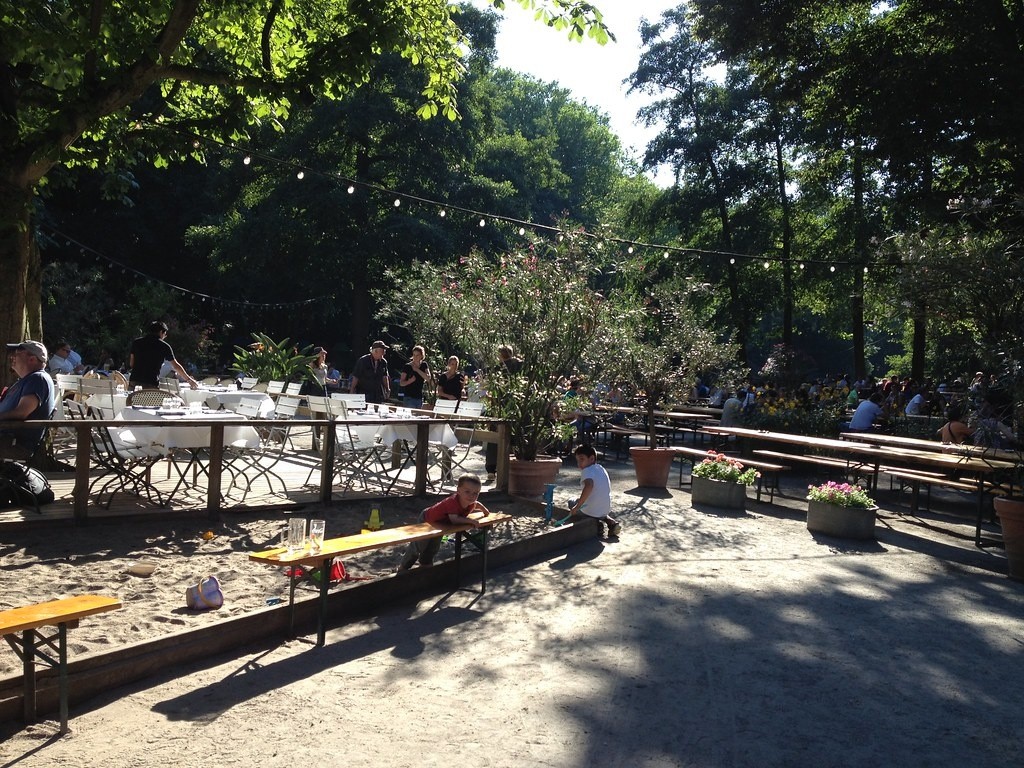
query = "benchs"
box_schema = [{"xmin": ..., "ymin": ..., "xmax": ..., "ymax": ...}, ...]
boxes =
[
  {"xmin": 600, "ymin": 421, "xmax": 1024, "ymax": 528},
  {"xmin": 0, "ymin": 594, "xmax": 124, "ymax": 735},
  {"xmin": 248, "ymin": 511, "xmax": 512, "ymax": 648}
]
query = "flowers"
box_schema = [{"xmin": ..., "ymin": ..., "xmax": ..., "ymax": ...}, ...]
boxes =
[
  {"xmin": 806, "ymin": 481, "xmax": 877, "ymax": 509},
  {"xmin": 227, "ymin": 332, "xmax": 324, "ymax": 394},
  {"xmin": 692, "ymin": 449, "xmax": 761, "ymax": 486}
]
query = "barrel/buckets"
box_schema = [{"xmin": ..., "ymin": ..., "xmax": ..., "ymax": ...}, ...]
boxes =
[{"xmin": 186, "ymin": 575, "xmax": 225, "ymax": 610}]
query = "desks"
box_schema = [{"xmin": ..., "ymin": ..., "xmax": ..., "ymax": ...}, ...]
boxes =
[
  {"xmin": 334, "ymin": 410, "xmax": 459, "ymax": 496},
  {"xmin": 598, "ymin": 403, "xmax": 711, "ymax": 461},
  {"xmin": 840, "ymin": 432, "xmax": 990, "ymax": 513},
  {"xmin": 702, "ymin": 427, "xmax": 887, "ymax": 501},
  {"xmin": 85, "ymin": 389, "xmax": 186, "ymax": 463},
  {"xmin": 848, "ymin": 447, "xmax": 1024, "ymax": 547},
  {"xmin": 942, "ymin": 448, "xmax": 1024, "ymax": 524},
  {"xmin": 659, "ymin": 404, "xmax": 723, "ymax": 446},
  {"xmin": 179, "ymin": 385, "xmax": 276, "ymax": 455},
  {"xmin": 106, "ymin": 405, "xmax": 263, "ymax": 505}
]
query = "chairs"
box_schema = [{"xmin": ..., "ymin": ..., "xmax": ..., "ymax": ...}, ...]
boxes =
[{"xmin": 14, "ymin": 374, "xmax": 483, "ymax": 509}]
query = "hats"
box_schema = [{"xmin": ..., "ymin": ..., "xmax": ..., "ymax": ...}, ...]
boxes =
[
  {"xmin": 7, "ymin": 339, "xmax": 48, "ymax": 363},
  {"xmin": 372, "ymin": 340, "xmax": 389, "ymax": 349},
  {"xmin": 311, "ymin": 347, "xmax": 327, "ymax": 355}
]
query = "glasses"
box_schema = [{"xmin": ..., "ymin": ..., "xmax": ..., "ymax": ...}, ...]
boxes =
[
  {"xmin": 60, "ymin": 347, "xmax": 70, "ymax": 354},
  {"xmin": 163, "ymin": 331, "xmax": 167, "ymax": 338}
]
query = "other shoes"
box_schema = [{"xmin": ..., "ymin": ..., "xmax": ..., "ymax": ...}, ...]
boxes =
[
  {"xmin": 598, "ymin": 522, "xmax": 604, "ymax": 536},
  {"xmin": 607, "ymin": 522, "xmax": 620, "ymax": 536}
]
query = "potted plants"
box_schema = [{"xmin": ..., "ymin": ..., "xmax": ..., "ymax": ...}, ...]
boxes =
[
  {"xmin": 580, "ymin": 303, "xmax": 731, "ymax": 488},
  {"xmin": 963, "ymin": 169, "xmax": 1024, "ymax": 584},
  {"xmin": 369, "ymin": 214, "xmax": 752, "ymax": 495}
]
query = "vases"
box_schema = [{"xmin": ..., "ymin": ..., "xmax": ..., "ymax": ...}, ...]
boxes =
[
  {"xmin": 249, "ymin": 417, "xmax": 288, "ymax": 443},
  {"xmin": 807, "ymin": 499, "xmax": 881, "ymax": 540},
  {"xmin": 691, "ymin": 474, "xmax": 747, "ymax": 511}
]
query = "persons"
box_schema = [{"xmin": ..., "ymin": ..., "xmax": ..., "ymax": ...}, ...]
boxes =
[
  {"xmin": 95, "ymin": 344, "xmax": 121, "ymax": 373},
  {"xmin": 127, "ymin": 318, "xmax": 198, "ymax": 393},
  {"xmin": 0, "ymin": 340, "xmax": 56, "ymax": 462},
  {"xmin": 568, "ymin": 443, "xmax": 620, "ymax": 537},
  {"xmin": 489, "ymin": 345, "xmax": 525, "ymax": 384},
  {"xmin": 40, "ymin": 334, "xmax": 90, "ymax": 376},
  {"xmin": 718, "ymin": 369, "xmax": 1024, "ymax": 495},
  {"xmin": 238, "ymin": 338, "xmax": 342, "ymax": 397},
  {"xmin": 396, "ymin": 473, "xmax": 490, "ymax": 578},
  {"xmin": 436, "ymin": 356, "xmax": 468, "ymax": 405},
  {"xmin": 400, "ymin": 343, "xmax": 434, "ymax": 408},
  {"xmin": 547, "ymin": 349, "xmax": 710, "ymax": 443},
  {"xmin": 350, "ymin": 339, "xmax": 391, "ymax": 402}
]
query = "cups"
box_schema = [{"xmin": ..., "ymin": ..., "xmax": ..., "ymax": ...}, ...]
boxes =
[
  {"xmin": 162, "ymin": 396, "xmax": 181, "ymax": 409},
  {"xmin": 280, "ymin": 518, "xmax": 306, "ymax": 554},
  {"xmin": 309, "ymin": 519, "xmax": 325, "ymax": 555},
  {"xmin": 134, "ymin": 385, "xmax": 143, "ymax": 392},
  {"xmin": 116, "ymin": 385, "xmax": 124, "ymax": 396},
  {"xmin": 189, "ymin": 401, "xmax": 203, "ymax": 414}
]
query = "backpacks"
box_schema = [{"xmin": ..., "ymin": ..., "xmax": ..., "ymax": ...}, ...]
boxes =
[{"xmin": 0, "ymin": 457, "xmax": 54, "ymax": 515}]
